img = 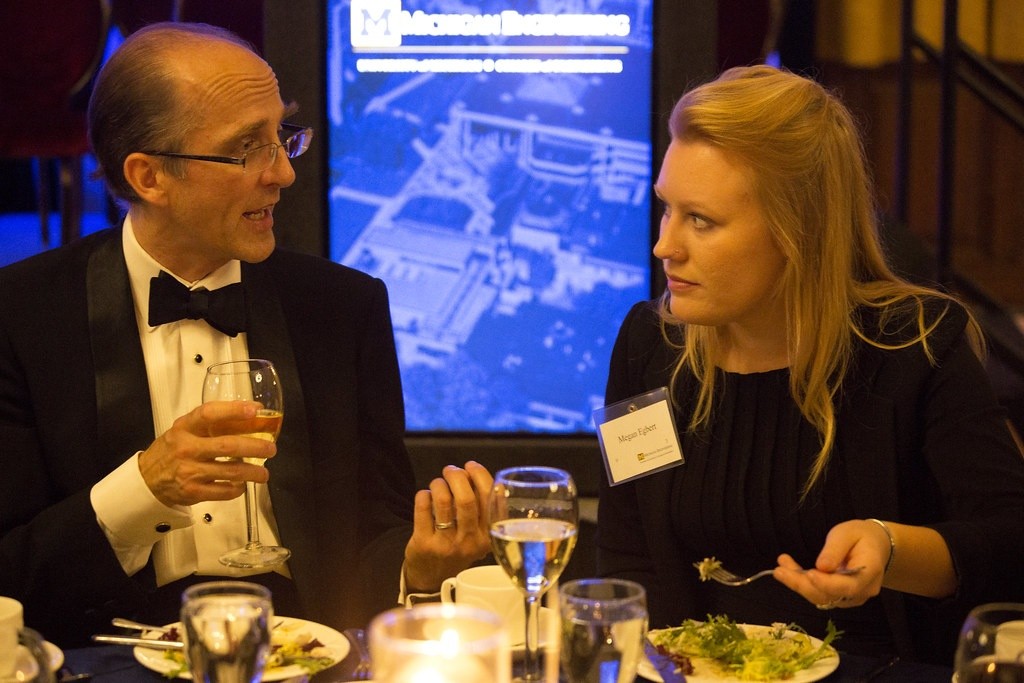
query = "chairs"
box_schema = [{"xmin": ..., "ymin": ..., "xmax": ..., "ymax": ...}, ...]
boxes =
[{"xmin": 1, "ymin": 0, "xmax": 105, "ymax": 248}]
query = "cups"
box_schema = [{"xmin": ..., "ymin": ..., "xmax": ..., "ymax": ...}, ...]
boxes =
[
  {"xmin": 0, "ymin": 596, "xmax": 23, "ymax": 676},
  {"xmin": 367, "ymin": 603, "xmax": 512, "ymax": 681},
  {"xmin": 558, "ymin": 578, "xmax": 648, "ymax": 683},
  {"xmin": 951, "ymin": 603, "xmax": 1024, "ymax": 683},
  {"xmin": 995, "ymin": 620, "xmax": 1024, "ymax": 663},
  {"xmin": 441, "ymin": 565, "xmax": 524, "ymax": 646},
  {"xmin": 184, "ymin": 581, "xmax": 271, "ymax": 683}
]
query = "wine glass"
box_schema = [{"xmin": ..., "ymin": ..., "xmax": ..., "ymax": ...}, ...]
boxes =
[
  {"xmin": 486, "ymin": 467, "xmax": 578, "ymax": 683},
  {"xmin": 202, "ymin": 359, "xmax": 290, "ymax": 568}
]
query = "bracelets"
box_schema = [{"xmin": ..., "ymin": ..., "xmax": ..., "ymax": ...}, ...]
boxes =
[{"xmin": 866, "ymin": 518, "xmax": 895, "ymax": 577}]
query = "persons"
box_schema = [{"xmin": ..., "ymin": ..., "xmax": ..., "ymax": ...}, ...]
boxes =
[
  {"xmin": 597, "ymin": 64, "xmax": 1024, "ymax": 666},
  {"xmin": 0, "ymin": 22, "xmax": 510, "ymax": 665}
]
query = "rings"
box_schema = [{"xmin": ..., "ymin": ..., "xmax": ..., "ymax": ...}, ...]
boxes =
[
  {"xmin": 815, "ymin": 595, "xmax": 845, "ymax": 610},
  {"xmin": 436, "ymin": 521, "xmax": 454, "ymax": 529}
]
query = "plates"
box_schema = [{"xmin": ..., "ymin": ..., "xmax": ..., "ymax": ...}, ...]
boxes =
[
  {"xmin": 512, "ymin": 607, "xmax": 549, "ymax": 661},
  {"xmin": 0, "ymin": 640, "xmax": 64, "ymax": 683},
  {"xmin": 134, "ymin": 615, "xmax": 350, "ymax": 683},
  {"xmin": 637, "ymin": 624, "xmax": 839, "ymax": 683}
]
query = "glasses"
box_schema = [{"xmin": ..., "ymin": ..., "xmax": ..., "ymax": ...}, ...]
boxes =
[{"xmin": 133, "ymin": 122, "xmax": 314, "ymax": 175}]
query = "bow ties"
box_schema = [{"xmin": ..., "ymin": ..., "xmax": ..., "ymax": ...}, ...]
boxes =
[{"xmin": 148, "ymin": 269, "xmax": 250, "ymax": 339}]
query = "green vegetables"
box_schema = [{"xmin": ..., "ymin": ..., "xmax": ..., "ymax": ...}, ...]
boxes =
[
  {"xmin": 161, "ymin": 645, "xmax": 335, "ymax": 682},
  {"xmin": 646, "ymin": 557, "xmax": 845, "ymax": 683}
]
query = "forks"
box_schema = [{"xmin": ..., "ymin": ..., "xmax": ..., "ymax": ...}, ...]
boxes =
[{"xmin": 694, "ymin": 561, "xmax": 866, "ymax": 586}]
